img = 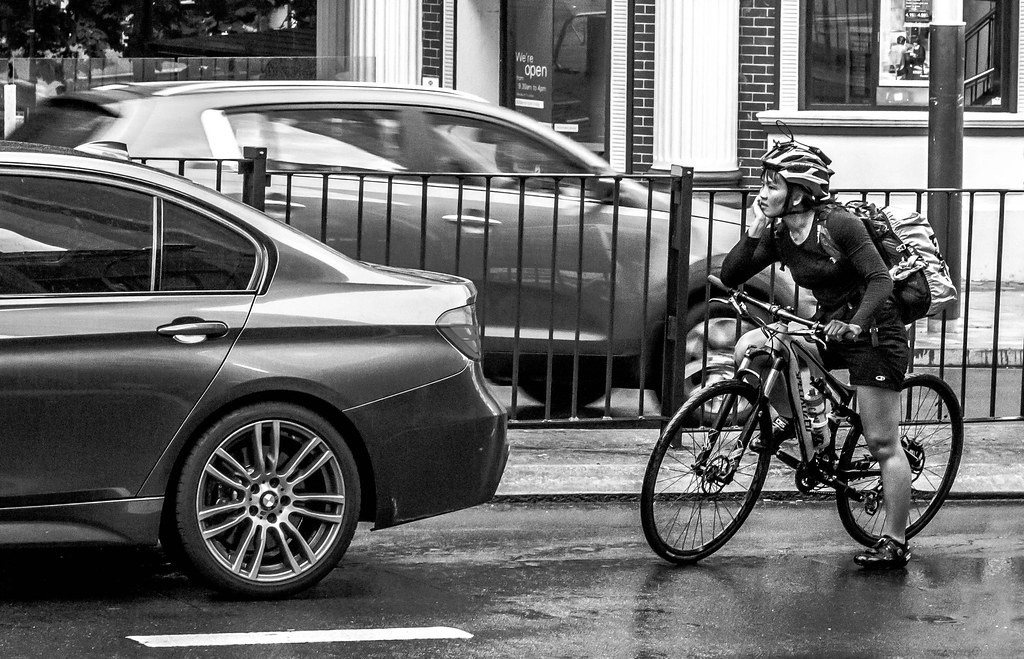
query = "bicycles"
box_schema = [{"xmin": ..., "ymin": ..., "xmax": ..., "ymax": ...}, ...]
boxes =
[{"xmin": 639, "ymin": 273, "xmax": 966, "ymax": 567}]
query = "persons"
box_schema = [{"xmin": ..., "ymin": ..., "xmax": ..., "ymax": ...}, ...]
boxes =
[{"xmin": 720, "ymin": 144, "xmax": 913, "ymax": 570}]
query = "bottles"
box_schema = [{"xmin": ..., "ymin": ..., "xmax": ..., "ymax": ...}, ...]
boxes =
[{"xmin": 803, "ymin": 388, "xmax": 831, "ymax": 449}]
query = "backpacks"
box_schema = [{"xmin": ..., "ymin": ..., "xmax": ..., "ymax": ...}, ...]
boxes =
[{"xmin": 817, "ymin": 199, "xmax": 960, "ymax": 326}]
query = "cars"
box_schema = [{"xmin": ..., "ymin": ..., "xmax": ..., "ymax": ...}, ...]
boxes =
[{"xmin": 0, "ymin": 139, "xmax": 511, "ymax": 603}]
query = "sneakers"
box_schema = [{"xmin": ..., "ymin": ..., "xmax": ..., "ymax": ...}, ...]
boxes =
[
  {"xmin": 853, "ymin": 535, "xmax": 913, "ymax": 571},
  {"xmin": 748, "ymin": 415, "xmax": 798, "ymax": 453}
]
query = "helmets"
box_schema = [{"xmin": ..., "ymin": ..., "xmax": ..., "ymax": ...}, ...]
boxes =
[{"xmin": 759, "ymin": 141, "xmax": 830, "ymax": 199}]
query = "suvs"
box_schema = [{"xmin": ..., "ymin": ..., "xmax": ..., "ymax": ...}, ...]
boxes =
[{"xmin": 0, "ymin": 78, "xmax": 819, "ymax": 422}]
